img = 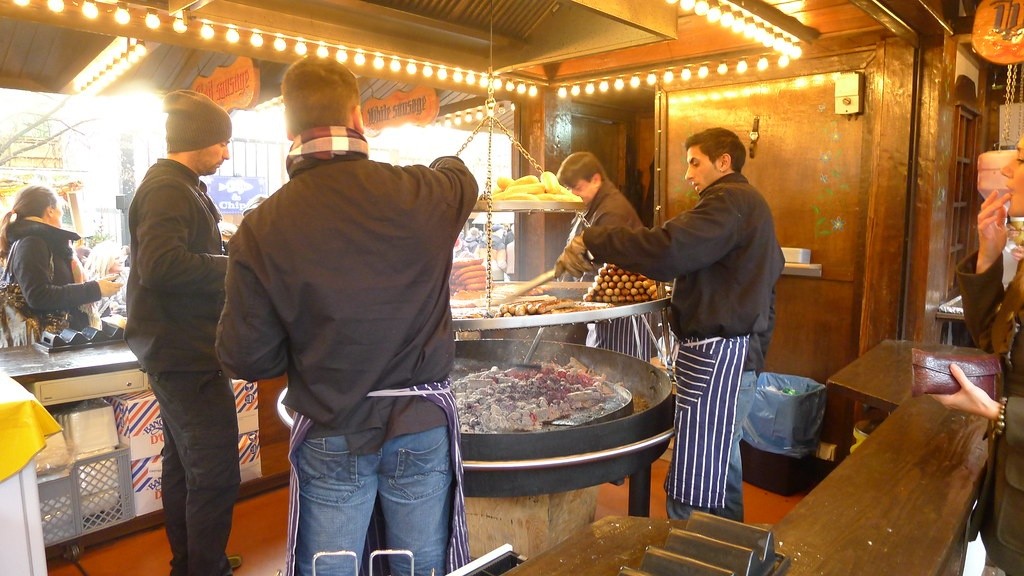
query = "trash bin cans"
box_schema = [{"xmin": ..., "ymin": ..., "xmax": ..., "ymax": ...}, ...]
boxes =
[{"xmin": 738, "ymin": 371, "xmax": 827, "ymax": 496}]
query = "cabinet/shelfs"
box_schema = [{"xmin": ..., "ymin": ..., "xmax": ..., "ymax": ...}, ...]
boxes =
[{"xmin": 944, "ymin": 99, "xmax": 983, "ymax": 302}]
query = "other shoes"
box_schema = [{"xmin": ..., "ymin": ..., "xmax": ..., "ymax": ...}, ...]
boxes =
[{"xmin": 227, "ymin": 556, "xmax": 242, "ymax": 569}]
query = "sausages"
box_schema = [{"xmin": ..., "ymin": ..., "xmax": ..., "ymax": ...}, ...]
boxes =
[
  {"xmin": 498, "ymin": 296, "xmax": 574, "ymax": 317},
  {"xmin": 449, "ymin": 257, "xmax": 487, "ymax": 295},
  {"xmin": 582, "ymin": 263, "xmax": 660, "ymax": 304}
]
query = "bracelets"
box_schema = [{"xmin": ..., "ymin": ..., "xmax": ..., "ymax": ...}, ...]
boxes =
[{"xmin": 989, "ymin": 395, "xmax": 1007, "ymax": 441}]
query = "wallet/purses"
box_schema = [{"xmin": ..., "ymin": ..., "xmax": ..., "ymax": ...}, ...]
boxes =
[{"xmin": 911, "ymin": 348, "xmax": 1001, "ymax": 400}]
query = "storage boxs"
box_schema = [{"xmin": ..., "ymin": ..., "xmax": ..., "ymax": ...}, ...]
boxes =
[{"xmin": 38, "ymin": 380, "xmax": 264, "ymax": 549}]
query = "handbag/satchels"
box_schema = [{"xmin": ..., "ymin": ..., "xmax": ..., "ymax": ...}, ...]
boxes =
[{"xmin": 0, "ymin": 238, "xmax": 70, "ymax": 348}]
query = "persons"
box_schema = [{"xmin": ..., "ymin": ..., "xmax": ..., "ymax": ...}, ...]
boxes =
[
  {"xmin": 123, "ymin": 88, "xmax": 240, "ymax": 576},
  {"xmin": 556, "ymin": 152, "xmax": 652, "ymax": 364},
  {"xmin": 553, "ymin": 126, "xmax": 786, "ymax": 523},
  {"xmin": 455, "ymin": 222, "xmax": 515, "ymax": 282},
  {"xmin": 923, "ymin": 122, "xmax": 1024, "ymax": 576},
  {"xmin": 214, "ymin": 54, "xmax": 479, "ymax": 576},
  {"xmin": 1, "ymin": 186, "xmax": 123, "ymax": 345}
]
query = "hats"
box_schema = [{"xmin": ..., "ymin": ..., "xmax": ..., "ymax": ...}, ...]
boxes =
[{"xmin": 162, "ymin": 89, "xmax": 232, "ymax": 153}]
